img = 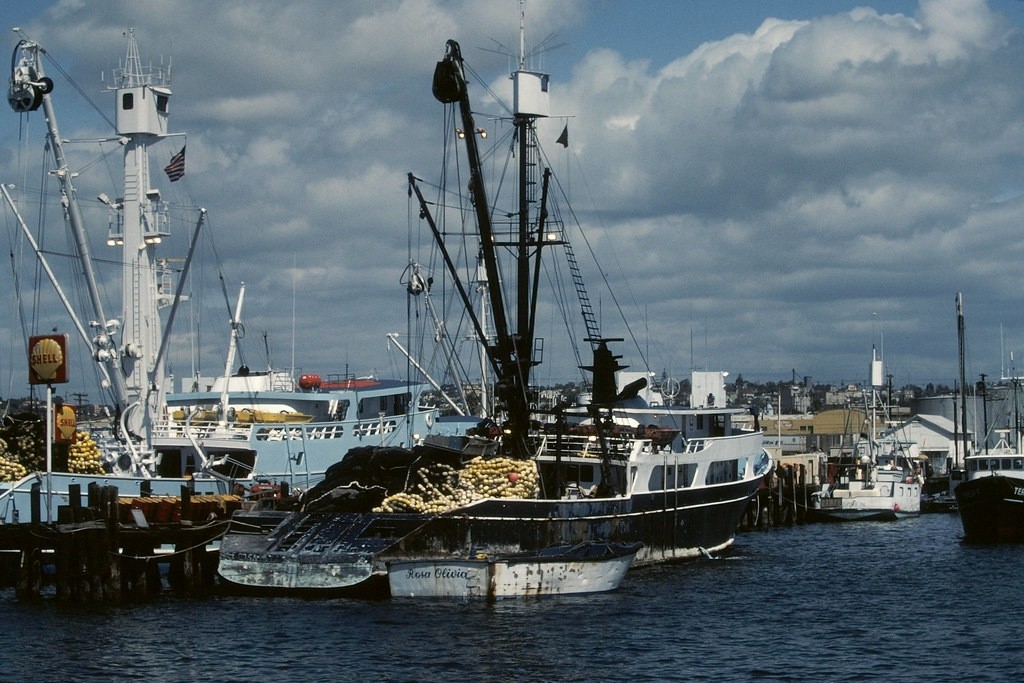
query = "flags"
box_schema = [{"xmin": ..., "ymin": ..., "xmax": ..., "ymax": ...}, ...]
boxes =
[
  {"xmin": 164, "ymin": 145, "xmax": 185, "ymax": 182},
  {"xmin": 556, "ymin": 125, "xmax": 569, "ymax": 148}
]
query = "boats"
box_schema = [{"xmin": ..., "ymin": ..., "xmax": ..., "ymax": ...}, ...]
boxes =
[
  {"xmin": 809, "ymin": 328, "xmax": 928, "ymax": 521},
  {"xmin": 952, "ymin": 291, "xmax": 1024, "ymax": 537},
  {"xmin": 925, "ymin": 496, "xmax": 959, "ymax": 513},
  {"xmin": 0, "ymin": 9, "xmax": 778, "ymax": 600}
]
